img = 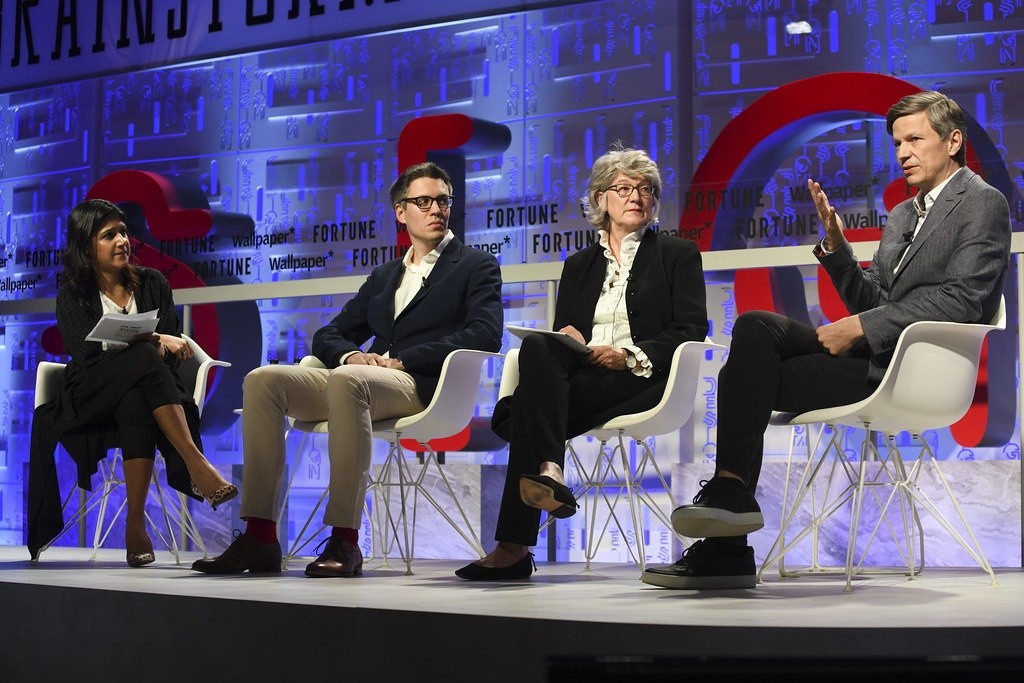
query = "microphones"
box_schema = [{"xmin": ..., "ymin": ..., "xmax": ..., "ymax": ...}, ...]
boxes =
[
  {"xmin": 122, "ymin": 307, "xmax": 128, "ymax": 315},
  {"xmin": 904, "ymin": 231, "xmax": 914, "ymax": 242},
  {"xmin": 627, "ymin": 270, "xmax": 634, "ymax": 281},
  {"xmin": 422, "ymin": 276, "xmax": 429, "ymax": 288}
]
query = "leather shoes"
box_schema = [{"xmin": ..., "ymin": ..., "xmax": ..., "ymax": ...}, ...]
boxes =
[
  {"xmin": 306, "ymin": 534, "xmax": 363, "ymax": 577},
  {"xmin": 192, "ymin": 528, "xmax": 282, "ymax": 574}
]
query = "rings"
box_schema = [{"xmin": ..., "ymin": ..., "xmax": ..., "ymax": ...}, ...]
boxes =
[{"xmin": 182, "ymin": 350, "xmax": 187, "ymax": 356}]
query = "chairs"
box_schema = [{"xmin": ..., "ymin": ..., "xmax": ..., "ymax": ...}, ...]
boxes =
[
  {"xmin": 757, "ymin": 294, "xmax": 1008, "ymax": 592},
  {"xmin": 31, "ymin": 333, "xmax": 232, "ymax": 564},
  {"xmin": 234, "ymin": 349, "xmax": 505, "ymax": 575},
  {"xmin": 498, "ymin": 335, "xmax": 727, "ymax": 574}
]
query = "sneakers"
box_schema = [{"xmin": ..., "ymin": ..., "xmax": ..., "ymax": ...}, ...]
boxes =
[
  {"xmin": 641, "ymin": 540, "xmax": 756, "ymax": 590},
  {"xmin": 670, "ymin": 475, "xmax": 765, "ymax": 537}
]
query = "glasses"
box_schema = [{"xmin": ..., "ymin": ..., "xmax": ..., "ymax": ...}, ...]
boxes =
[
  {"xmin": 600, "ymin": 183, "xmax": 656, "ymax": 198},
  {"xmin": 395, "ymin": 194, "xmax": 456, "ymax": 209}
]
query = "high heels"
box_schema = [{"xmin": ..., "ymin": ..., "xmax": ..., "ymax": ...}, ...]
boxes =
[
  {"xmin": 125, "ymin": 516, "xmax": 155, "ymax": 566},
  {"xmin": 190, "ymin": 478, "xmax": 238, "ymax": 511}
]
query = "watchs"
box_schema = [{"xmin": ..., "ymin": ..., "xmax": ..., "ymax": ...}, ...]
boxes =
[{"xmin": 623, "ymin": 348, "xmax": 637, "ymax": 371}]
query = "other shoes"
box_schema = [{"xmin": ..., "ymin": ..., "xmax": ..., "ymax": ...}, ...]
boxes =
[
  {"xmin": 517, "ymin": 473, "xmax": 580, "ymax": 519},
  {"xmin": 454, "ymin": 550, "xmax": 537, "ymax": 582}
]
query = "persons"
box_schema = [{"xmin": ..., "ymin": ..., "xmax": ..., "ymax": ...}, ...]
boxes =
[
  {"xmin": 454, "ymin": 147, "xmax": 708, "ymax": 582},
  {"xmin": 189, "ymin": 161, "xmax": 503, "ymax": 577},
  {"xmin": 54, "ymin": 196, "xmax": 238, "ymax": 568},
  {"xmin": 636, "ymin": 87, "xmax": 1011, "ymax": 589}
]
